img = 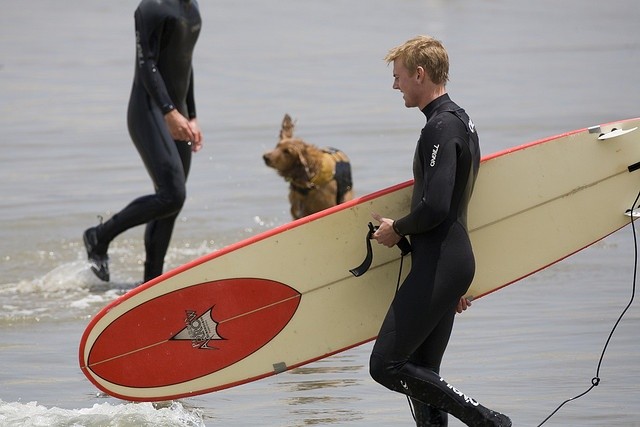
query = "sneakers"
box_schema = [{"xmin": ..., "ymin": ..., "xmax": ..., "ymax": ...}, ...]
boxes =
[{"xmin": 83, "ymin": 227, "xmax": 108, "ymax": 282}]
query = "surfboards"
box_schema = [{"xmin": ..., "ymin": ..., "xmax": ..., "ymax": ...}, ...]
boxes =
[{"xmin": 79, "ymin": 117, "xmax": 640, "ymax": 403}]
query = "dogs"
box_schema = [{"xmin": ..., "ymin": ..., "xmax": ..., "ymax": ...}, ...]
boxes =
[{"xmin": 262, "ymin": 113, "xmax": 354, "ymax": 220}]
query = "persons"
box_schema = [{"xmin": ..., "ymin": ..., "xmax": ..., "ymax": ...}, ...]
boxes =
[
  {"xmin": 368, "ymin": 36, "xmax": 512, "ymax": 426},
  {"xmin": 84, "ymin": 1, "xmax": 204, "ymax": 283}
]
